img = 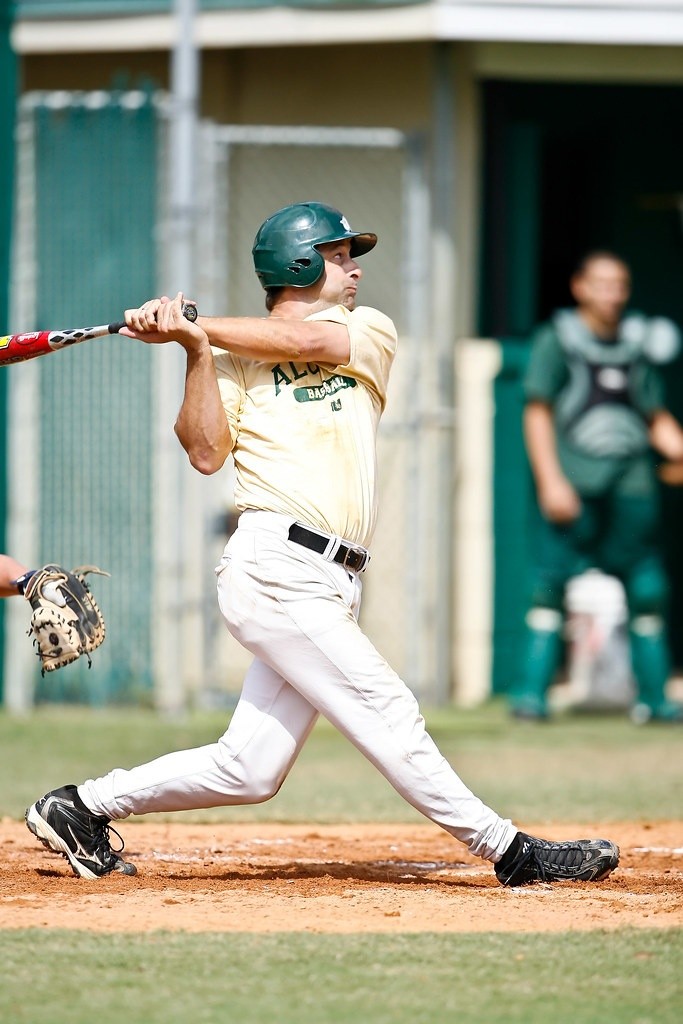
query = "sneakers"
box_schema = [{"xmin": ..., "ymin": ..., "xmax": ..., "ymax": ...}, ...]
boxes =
[
  {"xmin": 25, "ymin": 785, "xmax": 137, "ymax": 879},
  {"xmin": 495, "ymin": 832, "xmax": 620, "ymax": 887}
]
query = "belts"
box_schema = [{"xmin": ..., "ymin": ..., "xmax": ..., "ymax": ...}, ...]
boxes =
[{"xmin": 288, "ymin": 524, "xmax": 370, "ymax": 572}]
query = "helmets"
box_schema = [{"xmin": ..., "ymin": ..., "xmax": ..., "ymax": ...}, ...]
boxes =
[{"xmin": 253, "ymin": 200, "xmax": 378, "ymax": 288}]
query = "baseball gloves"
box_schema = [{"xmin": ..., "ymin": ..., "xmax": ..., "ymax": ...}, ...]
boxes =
[{"xmin": 9, "ymin": 563, "xmax": 113, "ymax": 679}]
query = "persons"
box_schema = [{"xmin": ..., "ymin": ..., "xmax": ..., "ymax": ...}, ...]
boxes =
[
  {"xmin": 28, "ymin": 201, "xmax": 620, "ymax": 886},
  {"xmin": 0, "ymin": 551, "xmax": 105, "ymax": 674},
  {"xmin": 524, "ymin": 247, "xmax": 683, "ymax": 725}
]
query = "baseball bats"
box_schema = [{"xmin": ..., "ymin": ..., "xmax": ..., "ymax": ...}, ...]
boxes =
[{"xmin": 0, "ymin": 303, "xmax": 199, "ymax": 367}]
